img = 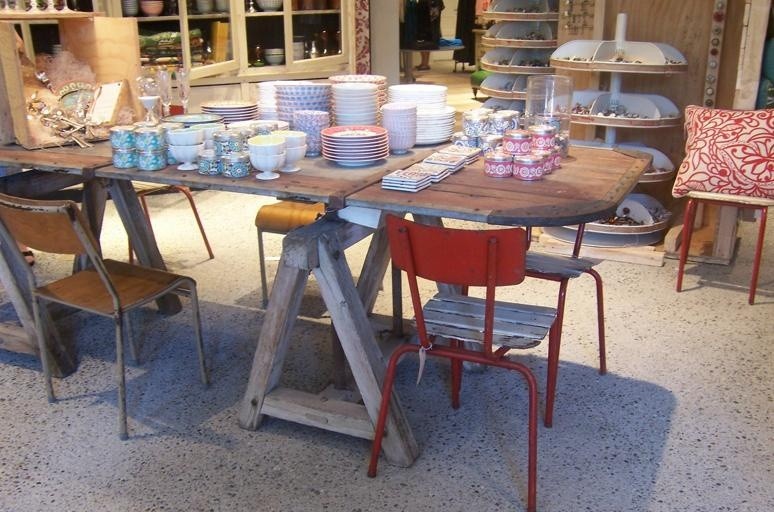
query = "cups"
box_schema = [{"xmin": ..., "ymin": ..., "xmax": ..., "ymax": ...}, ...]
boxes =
[{"xmin": 521, "ymin": 73, "xmax": 576, "ymax": 158}]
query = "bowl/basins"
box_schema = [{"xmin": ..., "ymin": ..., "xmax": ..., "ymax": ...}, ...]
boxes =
[
  {"xmin": 120, "ymin": 1, "xmax": 164, "ymax": 17},
  {"xmin": 195, "ymin": 0, "xmax": 229, "ymax": 14},
  {"xmin": 108, "ymin": 119, "xmax": 186, "ymax": 172},
  {"xmin": 380, "ymin": 105, "xmax": 418, "ymax": 156},
  {"xmin": 188, "ymin": 120, "xmax": 253, "ymax": 178},
  {"xmin": 451, "ymin": 106, "xmax": 522, "ymax": 156},
  {"xmin": 264, "ymin": 49, "xmax": 283, "ymax": 65},
  {"xmin": 253, "ymin": 110, "xmax": 329, "ymax": 158}
]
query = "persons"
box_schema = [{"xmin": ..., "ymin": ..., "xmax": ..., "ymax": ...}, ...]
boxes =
[
  {"xmin": 411, "ymin": 1, "xmax": 444, "ymax": 71},
  {"xmin": 13, "ymin": 239, "xmax": 35, "ymax": 268}
]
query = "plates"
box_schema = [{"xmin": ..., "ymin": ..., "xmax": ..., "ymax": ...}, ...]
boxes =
[
  {"xmin": 258, "ymin": 81, "xmax": 329, "ymax": 122},
  {"xmin": 162, "ymin": 102, "xmax": 258, "ymax": 126},
  {"xmin": 331, "ymin": 75, "xmax": 385, "ymax": 127},
  {"xmin": 321, "ymin": 127, "xmax": 390, "ymax": 166},
  {"xmin": 389, "ymin": 84, "xmax": 455, "ymax": 143}
]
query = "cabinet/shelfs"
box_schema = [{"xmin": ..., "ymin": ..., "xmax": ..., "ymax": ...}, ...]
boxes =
[
  {"xmin": 0, "ymin": 0, "xmax": 357, "ymax": 113},
  {"xmin": 540, "ymin": 14, "xmax": 689, "ymax": 246},
  {"xmin": 481, "ymin": 2, "xmax": 559, "ymax": 110}
]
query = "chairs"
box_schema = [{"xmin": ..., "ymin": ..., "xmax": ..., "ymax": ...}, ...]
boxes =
[
  {"xmin": 365, "ymin": 213, "xmax": 605, "ymax": 512},
  {"xmin": 1, "ymin": 181, "xmax": 213, "ymax": 440}
]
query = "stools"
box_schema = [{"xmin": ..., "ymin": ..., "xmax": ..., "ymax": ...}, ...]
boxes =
[
  {"xmin": 676, "ymin": 190, "xmax": 774, "ymax": 305},
  {"xmin": 255, "ymin": 198, "xmax": 327, "ymax": 311}
]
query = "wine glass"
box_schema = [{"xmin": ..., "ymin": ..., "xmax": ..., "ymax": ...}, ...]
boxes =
[
  {"xmin": 176, "ymin": 68, "xmax": 190, "ymax": 114},
  {"xmin": 159, "ymin": 71, "xmax": 172, "ymax": 117},
  {"xmin": 245, "ymin": 128, "xmax": 310, "ymax": 182},
  {"xmin": 167, "ymin": 127, "xmax": 206, "ymax": 171}
]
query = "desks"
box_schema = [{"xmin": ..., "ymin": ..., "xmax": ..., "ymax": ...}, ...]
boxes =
[{"xmin": 0, "ymin": 124, "xmax": 655, "ymax": 467}]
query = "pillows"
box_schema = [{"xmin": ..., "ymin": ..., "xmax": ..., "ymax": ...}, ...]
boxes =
[{"xmin": 671, "ymin": 104, "xmax": 774, "ymax": 200}]
